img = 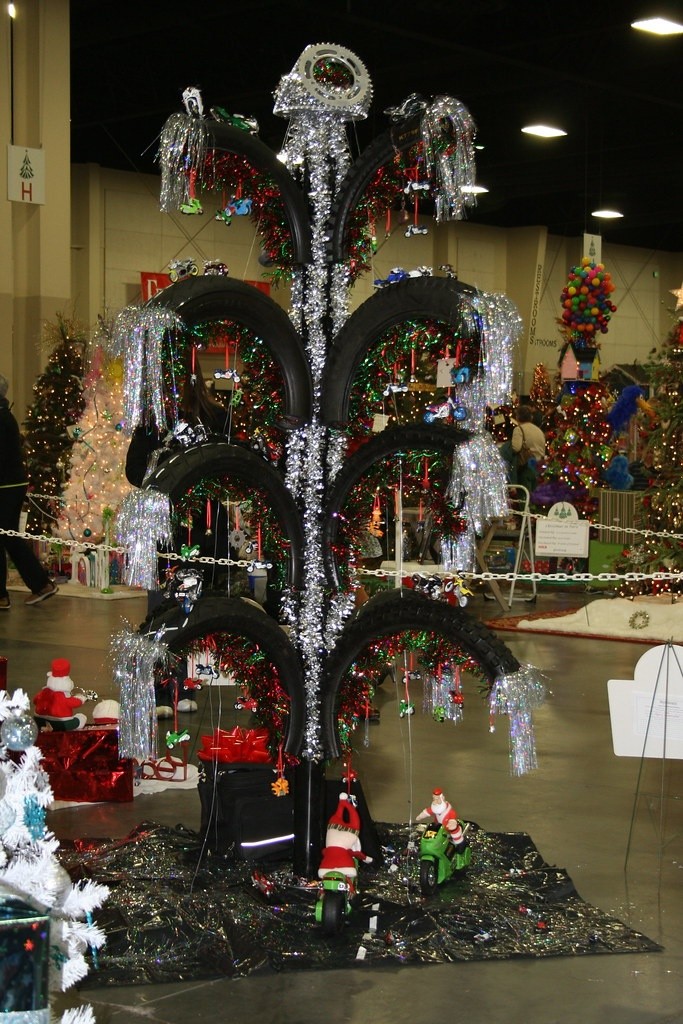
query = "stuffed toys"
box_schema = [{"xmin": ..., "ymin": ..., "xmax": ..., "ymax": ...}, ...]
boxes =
[
  {"xmin": 32, "ymin": 659, "xmax": 88, "ymax": 733},
  {"xmin": 317, "ymin": 792, "xmax": 373, "ymax": 894}
]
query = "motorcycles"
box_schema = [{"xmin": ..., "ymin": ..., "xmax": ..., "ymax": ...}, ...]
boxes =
[
  {"xmin": 313, "ymin": 840, "xmax": 359, "ymax": 937},
  {"xmin": 414, "ymin": 819, "xmax": 473, "ymax": 896}
]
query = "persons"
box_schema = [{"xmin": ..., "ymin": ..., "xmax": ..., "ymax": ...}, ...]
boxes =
[
  {"xmin": 417, "ymin": 788, "xmax": 467, "ymax": 855},
  {"xmin": 512, "ymin": 406, "xmax": 547, "ymax": 463},
  {"xmin": 0, "ymin": 375, "xmax": 60, "ymax": 609},
  {"xmin": 125, "ymin": 347, "xmax": 239, "ymax": 720}
]
